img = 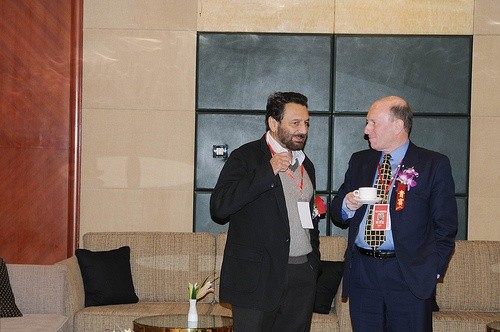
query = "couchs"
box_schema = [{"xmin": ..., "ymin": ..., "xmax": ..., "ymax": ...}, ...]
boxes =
[{"xmin": 0, "ymin": 231, "xmax": 500, "ymax": 332}]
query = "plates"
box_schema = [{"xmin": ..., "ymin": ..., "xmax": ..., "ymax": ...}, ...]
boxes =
[{"xmin": 354, "ymin": 197, "xmax": 383, "ymax": 205}]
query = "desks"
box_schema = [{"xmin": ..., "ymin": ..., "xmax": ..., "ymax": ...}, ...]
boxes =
[{"xmin": 133, "ymin": 314, "xmax": 233, "ymax": 332}]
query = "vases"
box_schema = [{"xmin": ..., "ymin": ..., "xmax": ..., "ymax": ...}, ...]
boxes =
[{"xmin": 188, "ymin": 299, "xmax": 198, "ymax": 328}]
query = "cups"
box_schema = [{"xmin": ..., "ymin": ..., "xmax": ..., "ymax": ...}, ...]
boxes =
[{"xmin": 353, "ymin": 186, "xmax": 377, "ymax": 199}]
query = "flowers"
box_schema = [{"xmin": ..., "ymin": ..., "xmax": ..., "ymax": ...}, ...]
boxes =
[
  {"xmin": 396, "ymin": 163, "xmax": 419, "ymax": 191},
  {"xmin": 313, "ymin": 195, "xmax": 325, "ymax": 220},
  {"xmin": 187, "ymin": 272, "xmax": 220, "ymax": 301}
]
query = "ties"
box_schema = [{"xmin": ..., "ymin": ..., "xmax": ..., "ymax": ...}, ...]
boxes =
[{"xmin": 364, "ymin": 154, "xmax": 392, "ymax": 251}]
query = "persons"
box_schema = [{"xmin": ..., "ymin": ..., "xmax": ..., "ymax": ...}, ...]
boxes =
[
  {"xmin": 329, "ymin": 96, "xmax": 458, "ymax": 332},
  {"xmin": 210, "ymin": 92, "xmax": 324, "ymax": 332}
]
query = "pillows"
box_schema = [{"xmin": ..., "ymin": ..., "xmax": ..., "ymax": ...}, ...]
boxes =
[
  {"xmin": 75, "ymin": 246, "xmax": 139, "ymax": 307},
  {"xmin": 0, "ymin": 257, "xmax": 23, "ymax": 318}
]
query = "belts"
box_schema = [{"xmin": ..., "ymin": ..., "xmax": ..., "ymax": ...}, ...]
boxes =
[{"xmin": 354, "ymin": 244, "xmax": 397, "ymax": 260}]
